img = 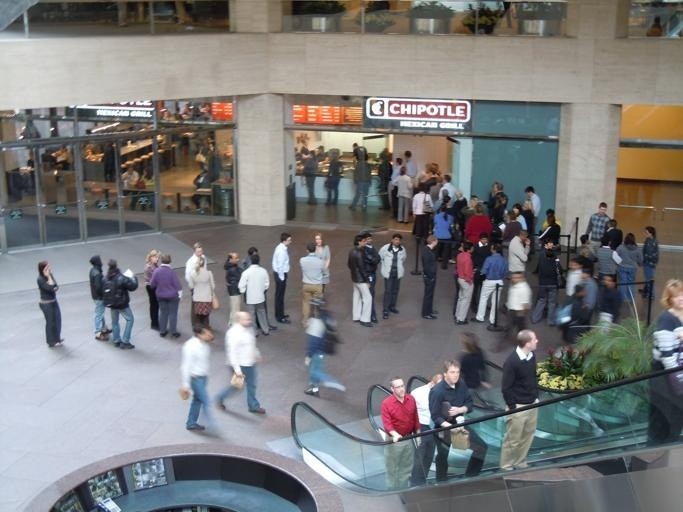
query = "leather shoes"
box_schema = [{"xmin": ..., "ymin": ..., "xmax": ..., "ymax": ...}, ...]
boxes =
[
  {"xmin": 359, "ymin": 321, "xmax": 373, "ymax": 328},
  {"xmin": 213, "ymin": 399, "xmax": 225, "ymax": 409},
  {"xmin": 187, "ymin": 424, "xmax": 205, "ymax": 431},
  {"xmin": 390, "ymin": 307, "xmax": 398, "ymax": 314},
  {"xmin": 384, "ymin": 311, "xmax": 389, "ymax": 319},
  {"xmin": 372, "ymin": 313, "xmax": 377, "ymax": 323},
  {"xmin": 267, "ymin": 325, "xmax": 277, "ymax": 330},
  {"xmin": 282, "ymin": 314, "xmax": 290, "ymax": 318},
  {"xmin": 431, "ymin": 309, "xmax": 439, "ymax": 314},
  {"xmin": 470, "ymin": 317, "xmax": 483, "ymax": 323},
  {"xmin": 642, "ymin": 294, "xmax": 654, "ymax": 299},
  {"xmin": 423, "ymin": 314, "xmax": 437, "ymax": 319},
  {"xmin": 639, "ymin": 289, "xmax": 648, "ymax": 294},
  {"xmin": 280, "ymin": 317, "xmax": 290, "ymax": 325},
  {"xmin": 457, "ymin": 320, "xmax": 469, "ymax": 325},
  {"xmin": 249, "ymin": 407, "xmax": 265, "ymax": 413}
]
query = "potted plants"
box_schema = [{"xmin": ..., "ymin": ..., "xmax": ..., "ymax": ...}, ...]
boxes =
[
  {"xmin": 516, "ymin": 2, "xmax": 563, "ymax": 35},
  {"xmin": 571, "ymin": 286, "xmax": 657, "ymax": 412},
  {"xmin": 311, "ymin": 0, "xmax": 345, "ymax": 32},
  {"xmin": 405, "ymin": 1, "xmax": 455, "ymax": 34},
  {"xmin": 355, "ymin": 8, "xmax": 397, "ymax": 32},
  {"xmin": 460, "ymin": 3, "xmax": 503, "ymax": 34}
]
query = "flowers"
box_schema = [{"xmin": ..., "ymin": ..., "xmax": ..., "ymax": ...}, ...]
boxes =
[{"xmin": 536, "ymin": 347, "xmax": 586, "ymax": 390}]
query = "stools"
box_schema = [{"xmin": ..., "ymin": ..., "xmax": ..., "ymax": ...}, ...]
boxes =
[{"xmin": 120, "ymin": 149, "xmax": 165, "ymax": 176}]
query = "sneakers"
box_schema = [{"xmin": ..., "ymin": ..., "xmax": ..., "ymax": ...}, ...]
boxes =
[
  {"xmin": 303, "ymin": 385, "xmax": 319, "ymax": 395},
  {"xmin": 114, "ymin": 340, "xmax": 124, "ymax": 347},
  {"xmin": 100, "ymin": 327, "xmax": 112, "ymax": 333},
  {"xmin": 173, "ymin": 333, "xmax": 182, "ymax": 339},
  {"xmin": 59, "ymin": 339, "xmax": 64, "ymax": 343},
  {"xmin": 50, "ymin": 342, "xmax": 60, "ymax": 347},
  {"xmin": 160, "ymin": 329, "xmax": 169, "ymax": 337},
  {"xmin": 96, "ymin": 331, "xmax": 108, "ymax": 341},
  {"xmin": 121, "ymin": 343, "xmax": 135, "ymax": 349}
]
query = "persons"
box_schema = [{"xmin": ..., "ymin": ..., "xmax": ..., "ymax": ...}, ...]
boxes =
[
  {"xmin": 455, "ymin": 240, "xmax": 477, "ymax": 325},
  {"xmin": 412, "ymin": 182, "xmax": 433, "ymax": 245},
  {"xmin": 378, "ymin": 233, "xmax": 407, "ymax": 319},
  {"xmin": 500, "ymin": 330, "xmax": 538, "ymax": 472},
  {"xmin": 438, "ymin": 174, "xmax": 456, "ymax": 208},
  {"xmin": 520, "ymin": 200, "xmax": 534, "ymax": 262},
  {"xmin": 470, "ymin": 195, "xmax": 482, "ymax": 210},
  {"xmin": 213, "ymin": 311, "xmax": 266, "ymax": 416},
  {"xmin": 453, "ymin": 241, "xmax": 463, "ymax": 317},
  {"xmin": 391, "ymin": 167, "xmax": 414, "ymax": 224},
  {"xmin": 537, "ymin": 214, "xmax": 564, "ymax": 287},
  {"xmin": 103, "ymin": 141, "xmax": 117, "ymax": 182},
  {"xmin": 594, "ymin": 237, "xmax": 621, "ymax": 287},
  {"xmin": 648, "ymin": 279, "xmax": 683, "ymax": 448},
  {"xmin": 193, "ymin": 135, "xmax": 222, "ymax": 210},
  {"xmin": 112, "ymin": 164, "xmax": 140, "ymax": 211},
  {"xmin": 638, "ymin": 226, "xmax": 659, "ymax": 299},
  {"xmin": 488, "ymin": 181, "xmax": 499, "ymax": 218},
  {"xmin": 238, "ymin": 254, "xmax": 270, "ymax": 337},
  {"xmin": 272, "ymin": 232, "xmax": 292, "ymax": 325},
  {"xmin": 148, "ymin": 253, "xmax": 183, "ymax": 340},
  {"xmin": 575, "ymin": 247, "xmax": 598, "ymax": 279},
  {"xmin": 531, "ymin": 248, "xmax": 563, "ymax": 327},
  {"xmin": 532, "ymin": 209, "xmax": 561, "ymax": 274},
  {"xmin": 465, "ymin": 203, "xmax": 493, "ymax": 247},
  {"xmin": 378, "ymin": 148, "xmax": 393, "ymax": 162},
  {"xmin": 454, "ymin": 333, "xmax": 491, "ymax": 408},
  {"xmin": 162, "ymin": 102, "xmax": 224, "ymax": 134},
  {"xmin": 38, "ymin": 260, "xmax": 64, "ymax": 348},
  {"xmin": 297, "ymin": 148, "xmax": 318, "ymax": 206},
  {"xmin": 313, "ymin": 231, "xmax": 331, "ymax": 294},
  {"xmin": 314, "ymin": 146, "xmax": 328, "ymax": 166},
  {"xmin": 324, "ymin": 150, "xmax": 343, "ymax": 207},
  {"xmin": 421, "ymin": 235, "xmax": 439, "ymax": 320},
  {"xmin": 377, "ymin": 153, "xmax": 393, "ymax": 211},
  {"xmin": 432, "ymin": 205, "xmax": 454, "ymax": 269},
  {"xmin": 585, "ymin": 202, "xmax": 611, "ymax": 252},
  {"xmin": 185, "ymin": 242, "xmax": 207, "ymax": 333},
  {"xmin": 437, "ymin": 196, "xmax": 456, "ymax": 264},
  {"xmin": 563, "ymin": 285, "xmax": 592, "ymax": 343},
  {"xmin": 102, "ymin": 259, "xmax": 139, "ymax": 350},
  {"xmin": 477, "ymin": 184, "xmax": 509, "ymax": 219},
  {"xmin": 403, "ymin": 151, "xmax": 417, "ymax": 216},
  {"xmin": 347, "ymin": 234, "xmax": 374, "ymax": 328},
  {"xmin": 577, "ymin": 234, "xmax": 598, "ymax": 262},
  {"xmin": 428, "ymin": 360, "xmax": 488, "ymax": 483},
  {"xmin": 352, "ymin": 143, "xmax": 368, "ymax": 168},
  {"xmin": 597, "ymin": 275, "xmax": 622, "ymax": 328},
  {"xmin": 138, "ymin": 166, "xmax": 155, "ymax": 211},
  {"xmin": 499, "ymin": 230, "xmax": 531, "ymax": 316},
  {"xmin": 144, "ymin": 250, "xmax": 161, "ymax": 331},
  {"xmin": 224, "ymin": 252, "xmax": 244, "ymax": 329},
  {"xmin": 491, "ymin": 193, "xmax": 506, "ymax": 229},
  {"xmin": 382, "ymin": 378, "xmax": 421, "ymax": 493},
  {"xmin": 564, "ymin": 258, "xmax": 583, "ymax": 329},
  {"xmin": 409, "ymin": 374, "xmax": 443, "ymax": 485},
  {"xmin": 299, "ymin": 243, "xmax": 327, "ymax": 327},
  {"xmin": 575, "ymin": 266, "xmax": 599, "ymax": 329},
  {"xmin": 454, "ymin": 192, "xmax": 467, "ymax": 233},
  {"xmin": 179, "ymin": 324, "xmax": 221, "ymax": 431},
  {"xmin": 615, "ymin": 233, "xmax": 643, "ymax": 305},
  {"xmin": 241, "ymin": 247, "xmax": 277, "ymax": 331},
  {"xmin": 303, "ymin": 295, "xmax": 346, "ymax": 395},
  {"xmin": 460, "ymin": 199, "xmax": 487, "ymax": 222},
  {"xmin": 502, "ymin": 211, "xmax": 523, "ymax": 270},
  {"xmin": 470, "ymin": 243, "xmax": 507, "ymax": 325},
  {"xmin": 414, "ymin": 161, "xmax": 443, "ymax": 186},
  {"xmin": 524, "ymin": 186, "xmax": 541, "ymax": 255},
  {"xmin": 89, "ymin": 254, "xmax": 113, "ymax": 342},
  {"xmin": 362, "ymin": 231, "xmax": 381, "ymax": 323},
  {"xmin": 390, "ymin": 158, "xmax": 403, "ymax": 219},
  {"xmin": 504, "ymin": 203, "xmax": 527, "ymax": 231},
  {"xmin": 432, "ymin": 190, "xmax": 455, "ymax": 216},
  {"xmin": 188, "ymin": 256, "xmax": 215, "ymax": 331},
  {"xmin": 488, "ymin": 272, "xmax": 532, "ymax": 352},
  {"xmin": 52, "ymin": 145, "xmax": 72, "ymax": 170},
  {"xmin": 538, "ymin": 237, "xmax": 563, "ymax": 313},
  {"xmin": 348, "ymin": 152, "xmax": 372, "ymax": 214},
  {"xmin": 603, "ymin": 219, "xmax": 623, "ymax": 250},
  {"xmin": 471, "ymin": 231, "xmax": 492, "ymax": 314}
]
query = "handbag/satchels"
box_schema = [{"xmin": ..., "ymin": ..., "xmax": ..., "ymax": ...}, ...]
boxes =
[
  {"xmin": 555, "ymin": 256, "xmax": 566, "ymax": 289},
  {"xmin": 208, "ymin": 270, "xmax": 219, "ymax": 309},
  {"xmin": 423, "ymin": 193, "xmax": 433, "ymax": 214}
]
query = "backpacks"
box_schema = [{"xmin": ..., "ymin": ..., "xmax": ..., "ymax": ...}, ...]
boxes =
[
  {"xmin": 323, "ymin": 318, "xmax": 340, "ymax": 355},
  {"xmin": 103, "ymin": 274, "xmax": 122, "ymax": 308}
]
